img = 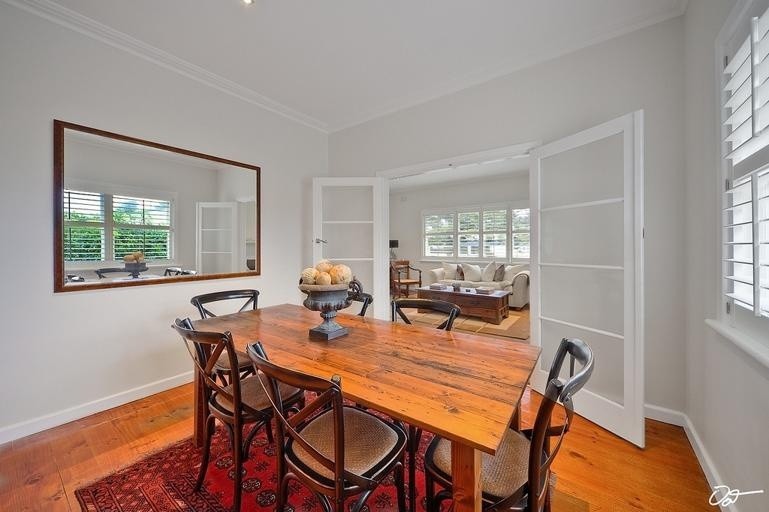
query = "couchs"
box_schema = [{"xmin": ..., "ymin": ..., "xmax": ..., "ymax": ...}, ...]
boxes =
[{"xmin": 430, "ymin": 268, "xmax": 530, "ymax": 311}]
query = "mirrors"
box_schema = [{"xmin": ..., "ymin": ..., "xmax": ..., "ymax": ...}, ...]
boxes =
[{"xmin": 54, "ymin": 119, "xmax": 261, "ymax": 293}]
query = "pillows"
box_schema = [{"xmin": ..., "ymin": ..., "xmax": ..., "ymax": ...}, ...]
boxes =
[{"xmin": 440, "ymin": 261, "xmax": 521, "ymax": 281}]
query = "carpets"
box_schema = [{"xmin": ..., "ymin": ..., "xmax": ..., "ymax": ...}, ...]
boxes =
[
  {"xmin": 73, "ymin": 389, "xmax": 455, "ymax": 511},
  {"xmin": 411, "ymin": 312, "xmax": 530, "ymax": 340}
]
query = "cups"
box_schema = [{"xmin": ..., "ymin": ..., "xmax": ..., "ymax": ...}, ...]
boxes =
[{"xmin": 453, "ymin": 283, "xmax": 461, "ymax": 291}]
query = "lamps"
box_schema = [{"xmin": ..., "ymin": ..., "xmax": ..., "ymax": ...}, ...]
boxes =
[{"xmin": 389, "ymin": 240, "xmax": 398, "ymax": 260}]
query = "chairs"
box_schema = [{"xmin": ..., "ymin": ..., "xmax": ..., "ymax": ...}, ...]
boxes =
[
  {"xmin": 355, "ymin": 298, "xmax": 462, "ymax": 451},
  {"xmin": 172, "ymin": 317, "xmax": 305, "ymax": 509},
  {"xmin": 64, "ymin": 268, "xmax": 197, "ymax": 286},
  {"xmin": 425, "ymin": 336, "xmax": 594, "ymax": 511},
  {"xmin": 345, "ymin": 291, "xmax": 373, "ymax": 316},
  {"xmin": 389, "ymin": 261, "xmax": 422, "ymax": 298},
  {"xmin": 245, "ymin": 341, "xmax": 421, "ymax": 511},
  {"xmin": 189, "ymin": 288, "xmax": 260, "ymax": 434}
]
